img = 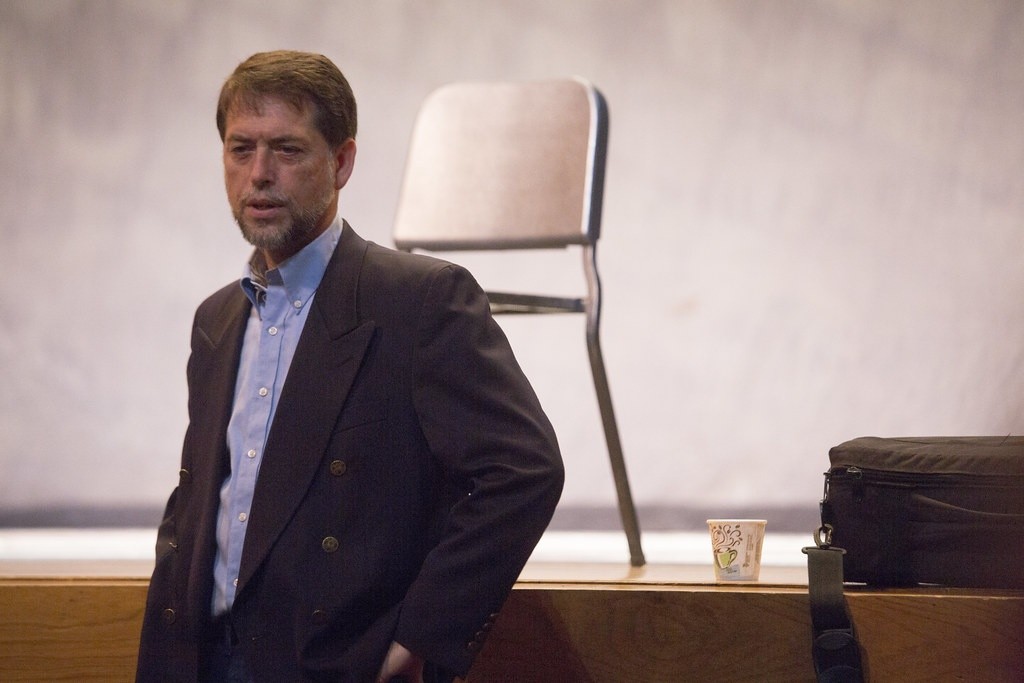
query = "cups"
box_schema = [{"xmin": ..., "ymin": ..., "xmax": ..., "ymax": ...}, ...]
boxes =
[{"xmin": 706, "ymin": 519, "xmax": 767, "ymax": 581}]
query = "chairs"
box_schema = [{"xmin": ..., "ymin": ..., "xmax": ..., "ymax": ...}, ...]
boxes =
[{"xmin": 391, "ymin": 77, "xmax": 647, "ymax": 568}]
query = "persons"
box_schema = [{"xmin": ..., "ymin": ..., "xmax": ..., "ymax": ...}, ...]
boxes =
[{"xmin": 135, "ymin": 51, "xmax": 564, "ymax": 683}]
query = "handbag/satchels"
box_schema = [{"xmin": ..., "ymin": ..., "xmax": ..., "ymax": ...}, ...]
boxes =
[{"xmin": 820, "ymin": 436, "xmax": 1024, "ymax": 587}]
